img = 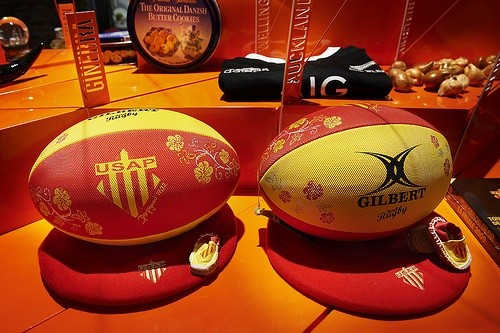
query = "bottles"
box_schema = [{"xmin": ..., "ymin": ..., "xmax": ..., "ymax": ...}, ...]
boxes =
[
  {"xmin": 9, "ymin": 30, "xmax": 20, "ymax": 45},
  {"xmin": 50, "ymin": 28, "xmax": 65, "ymax": 48}
]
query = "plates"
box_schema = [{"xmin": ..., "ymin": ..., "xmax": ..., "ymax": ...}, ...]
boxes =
[{"xmin": 143, "ymin": 26, "xmax": 180, "ymax": 57}]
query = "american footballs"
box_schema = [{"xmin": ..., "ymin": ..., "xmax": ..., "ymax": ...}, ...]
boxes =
[
  {"xmin": 256, "ymin": 103, "xmax": 453, "ymax": 241},
  {"xmin": 28, "ymin": 108, "xmax": 241, "ymax": 246}
]
